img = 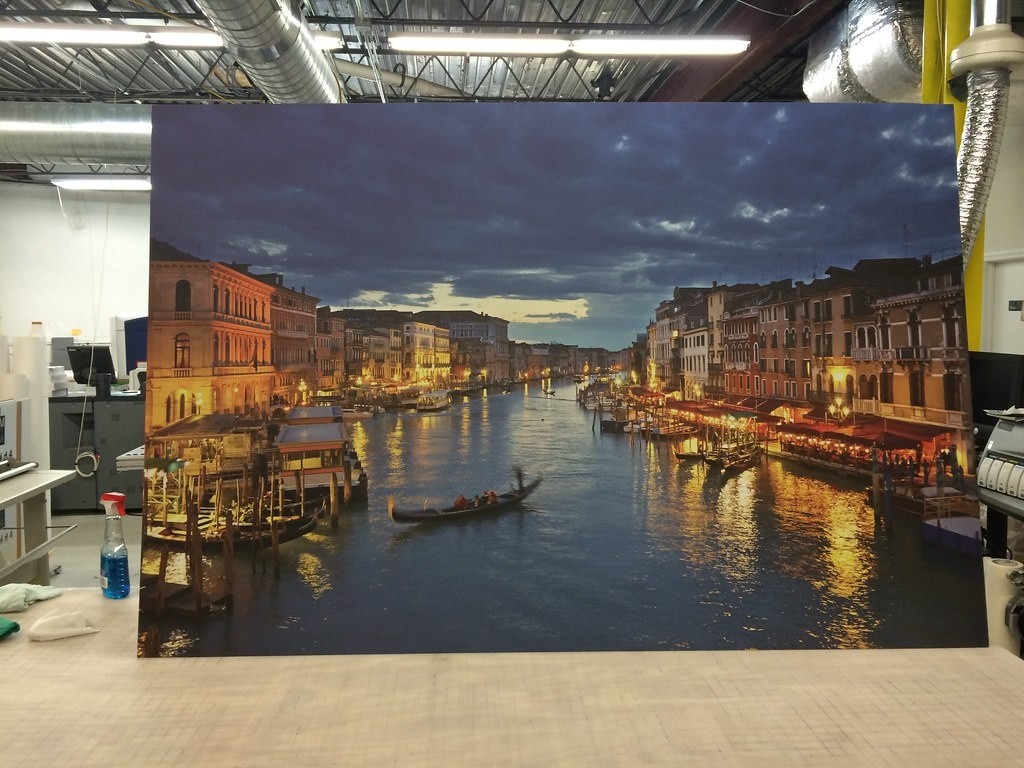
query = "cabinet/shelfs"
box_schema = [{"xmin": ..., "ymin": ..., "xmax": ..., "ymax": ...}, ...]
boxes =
[{"xmin": 50, "ymin": 387, "xmax": 144, "ymax": 512}]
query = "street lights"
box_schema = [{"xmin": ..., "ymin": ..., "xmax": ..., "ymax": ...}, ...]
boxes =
[{"xmin": 828, "ymin": 398, "xmax": 850, "ymax": 429}]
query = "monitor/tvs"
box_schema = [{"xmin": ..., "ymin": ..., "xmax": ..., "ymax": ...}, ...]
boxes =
[{"xmin": 66, "ymin": 346, "xmax": 119, "ymax": 384}]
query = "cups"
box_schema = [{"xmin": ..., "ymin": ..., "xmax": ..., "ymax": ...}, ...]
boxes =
[{"xmin": 96, "ymin": 373, "xmax": 111, "ymax": 396}]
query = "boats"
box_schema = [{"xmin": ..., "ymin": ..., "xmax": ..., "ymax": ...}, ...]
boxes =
[
  {"xmin": 341, "ymin": 409, "xmax": 373, "ymax": 418},
  {"xmin": 388, "ymin": 480, "xmax": 541, "ymax": 523},
  {"xmin": 353, "ymin": 404, "xmax": 385, "ymax": 414},
  {"xmin": 865, "ymin": 478, "xmax": 964, "ymax": 521},
  {"xmin": 272, "ymin": 405, "xmax": 370, "ymax": 500},
  {"xmin": 541, "ymin": 388, "xmax": 556, "ymax": 395},
  {"xmin": 145, "ymin": 464, "xmax": 328, "ymax": 546},
  {"xmin": 417, "ymin": 390, "xmax": 452, "ymax": 411},
  {"xmin": 573, "ymin": 375, "xmax": 765, "ymax": 471}
]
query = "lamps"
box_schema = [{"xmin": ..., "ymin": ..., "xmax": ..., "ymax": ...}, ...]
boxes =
[
  {"xmin": 389, "ymin": 31, "xmax": 753, "ymax": 58},
  {"xmin": 0, "ymin": 21, "xmax": 346, "ymax": 52}
]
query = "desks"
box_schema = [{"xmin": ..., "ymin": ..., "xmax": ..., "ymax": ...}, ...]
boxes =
[{"xmin": 0, "ymin": 469, "xmax": 79, "ymax": 585}]
